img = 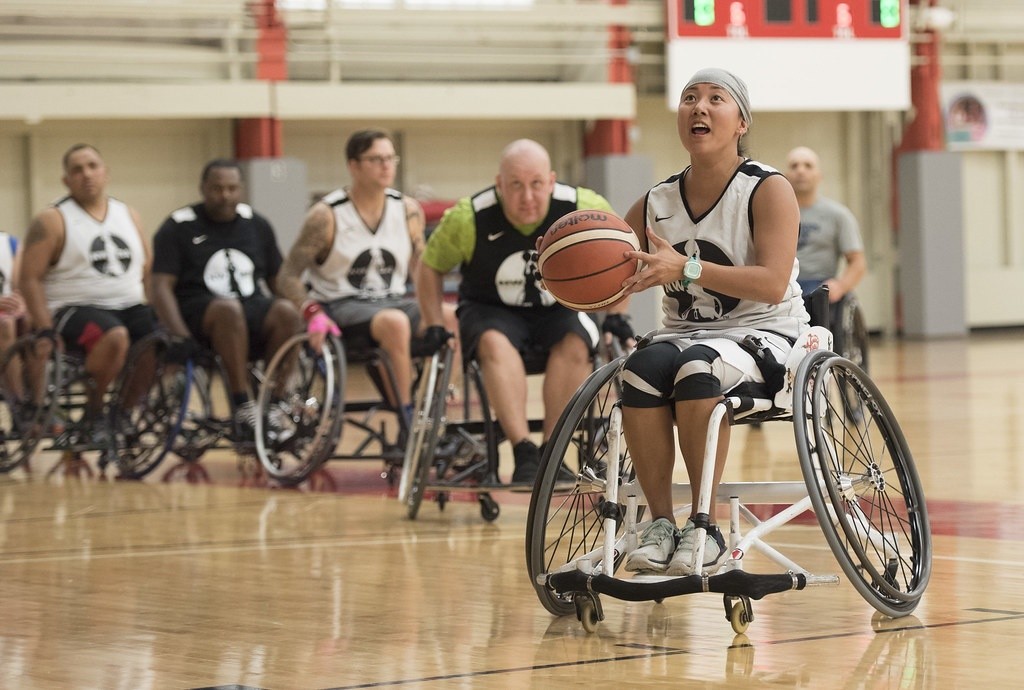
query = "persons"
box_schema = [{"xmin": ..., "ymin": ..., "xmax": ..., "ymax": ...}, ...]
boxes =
[
  {"xmin": 416, "ymin": 138, "xmax": 636, "ymax": 491},
  {"xmin": 785, "ymin": 143, "xmax": 867, "ymax": 300},
  {"xmin": 0, "ymin": 144, "xmax": 153, "ymax": 446},
  {"xmin": 160, "ymin": 155, "xmax": 301, "ymax": 444},
  {"xmin": 536, "ymin": 67, "xmax": 811, "ymax": 576},
  {"xmin": 279, "ymin": 126, "xmax": 459, "ymax": 448}
]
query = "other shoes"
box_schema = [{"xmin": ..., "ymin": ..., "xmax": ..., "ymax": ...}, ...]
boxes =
[
  {"xmin": 227, "ymin": 391, "xmax": 269, "ymax": 440},
  {"xmin": 539, "ymin": 442, "xmax": 578, "ymax": 488},
  {"xmin": 268, "ymin": 396, "xmax": 290, "ymax": 436},
  {"xmin": 510, "ymin": 439, "xmax": 537, "ymax": 490}
]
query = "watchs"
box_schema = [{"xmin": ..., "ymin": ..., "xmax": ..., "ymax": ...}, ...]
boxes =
[{"xmin": 683, "ymin": 257, "xmax": 702, "ymax": 282}]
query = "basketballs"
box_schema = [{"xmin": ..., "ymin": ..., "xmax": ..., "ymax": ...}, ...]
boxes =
[{"xmin": 530, "ymin": 204, "xmax": 649, "ymax": 318}]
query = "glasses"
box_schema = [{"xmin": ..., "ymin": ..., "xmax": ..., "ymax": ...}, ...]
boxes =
[{"xmin": 360, "ymin": 155, "xmax": 400, "ymax": 166}]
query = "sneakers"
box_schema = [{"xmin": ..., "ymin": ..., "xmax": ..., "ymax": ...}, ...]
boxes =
[
  {"xmin": 625, "ymin": 518, "xmax": 680, "ymax": 571},
  {"xmin": 668, "ymin": 518, "xmax": 731, "ymax": 575}
]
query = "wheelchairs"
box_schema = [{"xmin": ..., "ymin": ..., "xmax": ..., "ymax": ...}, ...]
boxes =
[
  {"xmin": 829, "ymin": 291, "xmax": 876, "ymax": 424},
  {"xmin": 0, "ymin": 316, "xmax": 276, "ymax": 483},
  {"xmin": 521, "ymin": 282, "xmax": 936, "ymax": 632},
  {"xmin": 250, "ymin": 323, "xmax": 417, "ymax": 487},
  {"xmin": 398, "ymin": 328, "xmax": 649, "ymax": 527}
]
